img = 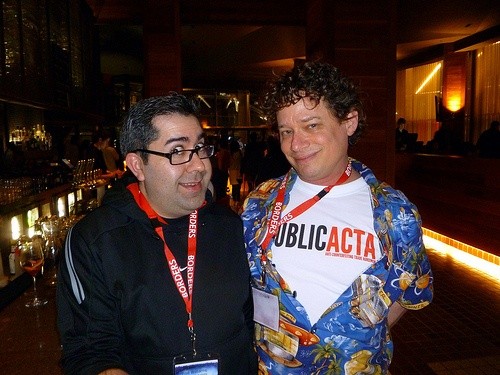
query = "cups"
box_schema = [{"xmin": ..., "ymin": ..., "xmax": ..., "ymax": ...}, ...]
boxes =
[{"xmin": 0, "ymin": 178, "xmax": 33, "ymax": 204}]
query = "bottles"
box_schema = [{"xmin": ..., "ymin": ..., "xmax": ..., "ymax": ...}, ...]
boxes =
[{"xmin": 8, "ymin": 243, "xmax": 15, "ymax": 276}]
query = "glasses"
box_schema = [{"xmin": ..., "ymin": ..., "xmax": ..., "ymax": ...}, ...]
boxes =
[{"xmin": 131, "ymin": 144, "xmax": 216, "ymax": 166}]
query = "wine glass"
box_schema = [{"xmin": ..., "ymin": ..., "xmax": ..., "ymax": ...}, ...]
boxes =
[{"xmin": 15, "ymin": 199, "xmax": 101, "ymax": 307}]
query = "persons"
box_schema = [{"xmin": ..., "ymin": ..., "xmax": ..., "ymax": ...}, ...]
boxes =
[
  {"xmin": 99, "ymin": 147, "xmax": 120, "ymax": 171},
  {"xmin": 395, "ymin": 117, "xmax": 412, "ymax": 153},
  {"xmin": 476, "ymin": 120, "xmax": 500, "ymax": 158},
  {"xmin": 93, "ymin": 133, "xmax": 112, "ymax": 174},
  {"xmin": 227, "ymin": 138, "xmax": 242, "ymax": 203},
  {"xmin": 241, "ymin": 60, "xmax": 434, "ymax": 375},
  {"xmin": 214, "ymin": 139, "xmax": 234, "ymax": 186},
  {"xmin": 254, "ymin": 127, "xmax": 270, "ymax": 189},
  {"xmin": 55, "ymin": 92, "xmax": 258, "ymax": 375},
  {"xmin": 435, "ymin": 119, "xmax": 461, "ymax": 146},
  {"xmin": 267, "ymin": 123, "xmax": 292, "ymax": 178}
]
